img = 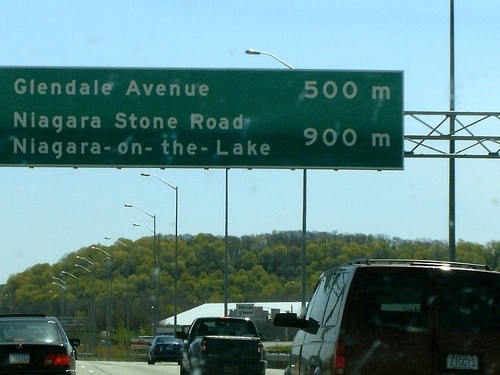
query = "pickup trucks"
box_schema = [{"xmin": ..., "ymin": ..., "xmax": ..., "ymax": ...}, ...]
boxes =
[{"xmin": 175, "ymin": 316, "xmax": 268, "ymax": 375}]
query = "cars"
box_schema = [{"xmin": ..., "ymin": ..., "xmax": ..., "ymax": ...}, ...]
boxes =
[
  {"xmin": 147, "ymin": 334, "xmax": 184, "ymax": 365},
  {"xmin": 0, "ymin": 312, "xmax": 81, "ymax": 375}
]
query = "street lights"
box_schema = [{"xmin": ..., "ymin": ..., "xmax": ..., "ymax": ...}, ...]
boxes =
[
  {"xmin": 244, "ymin": 48, "xmax": 307, "ymax": 318},
  {"xmin": 133, "ymin": 223, "xmax": 161, "ymax": 326},
  {"xmin": 76, "ymin": 255, "xmax": 97, "ymax": 333},
  {"xmin": 48, "ymin": 270, "xmax": 80, "ymax": 334},
  {"xmin": 104, "ymin": 236, "xmax": 130, "ymax": 357},
  {"xmin": 140, "ymin": 173, "xmax": 178, "ymax": 333},
  {"xmin": 91, "ymin": 246, "xmax": 113, "ymax": 345},
  {"xmin": 125, "ymin": 204, "xmax": 156, "ymax": 335}
]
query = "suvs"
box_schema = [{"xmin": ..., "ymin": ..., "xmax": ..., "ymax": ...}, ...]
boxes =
[{"xmin": 273, "ymin": 256, "xmax": 500, "ymax": 375}]
